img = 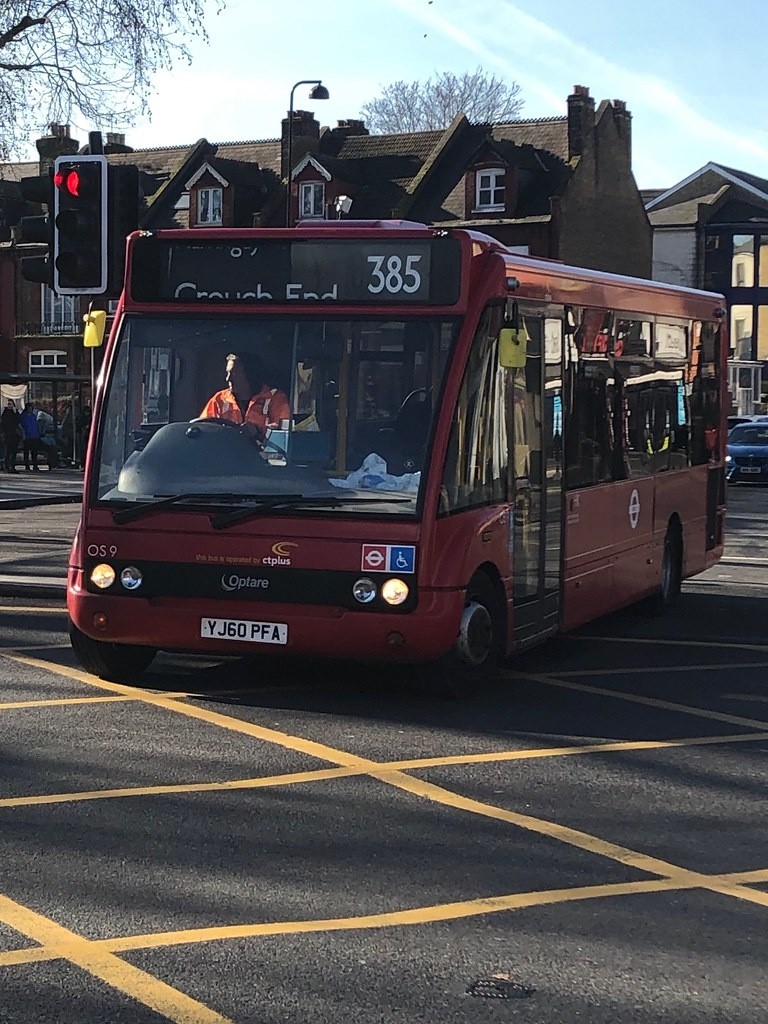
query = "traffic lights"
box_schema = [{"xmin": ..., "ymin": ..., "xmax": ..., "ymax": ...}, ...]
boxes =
[
  {"xmin": 54, "ymin": 154, "xmax": 108, "ymax": 294},
  {"xmin": 18, "ymin": 166, "xmax": 55, "ymax": 292}
]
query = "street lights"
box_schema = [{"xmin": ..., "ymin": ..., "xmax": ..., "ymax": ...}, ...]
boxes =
[{"xmin": 286, "ymin": 79, "xmax": 329, "ymax": 232}]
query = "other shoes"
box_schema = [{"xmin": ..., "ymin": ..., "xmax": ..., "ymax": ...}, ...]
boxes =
[
  {"xmin": 25, "ymin": 468, "xmax": 31, "ymax": 471},
  {"xmin": 5, "ymin": 468, "xmax": 19, "ymax": 474},
  {"xmin": 33, "ymin": 467, "xmax": 39, "ymax": 471}
]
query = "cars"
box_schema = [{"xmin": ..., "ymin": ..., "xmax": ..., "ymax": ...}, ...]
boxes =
[{"xmin": 725, "ymin": 414, "xmax": 768, "ymax": 484}]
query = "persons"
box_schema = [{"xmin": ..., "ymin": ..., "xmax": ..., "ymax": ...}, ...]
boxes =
[
  {"xmin": 189, "ymin": 348, "xmax": 291, "ymax": 437},
  {"xmin": 0, "ymin": 399, "xmax": 93, "ymax": 474}
]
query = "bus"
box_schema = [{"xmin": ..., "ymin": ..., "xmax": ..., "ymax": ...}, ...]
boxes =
[{"xmin": 64, "ymin": 213, "xmax": 736, "ymax": 700}]
